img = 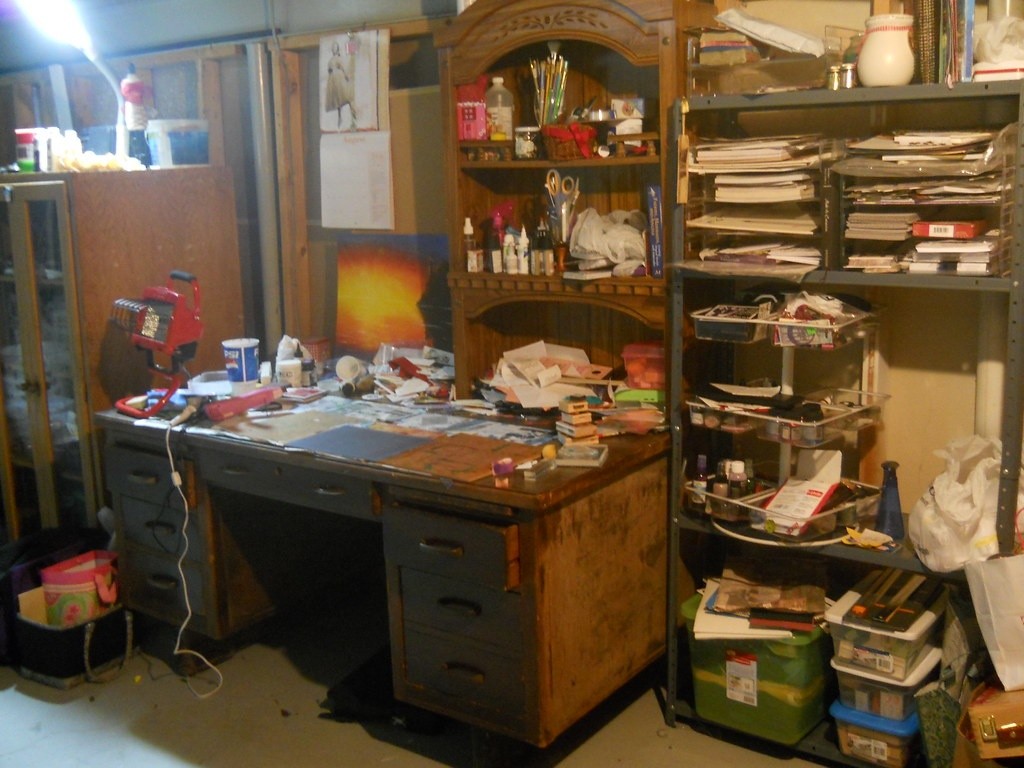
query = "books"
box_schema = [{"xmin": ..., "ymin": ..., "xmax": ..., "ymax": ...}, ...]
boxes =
[
  {"xmin": 842, "ymin": 570, "xmax": 943, "ymax": 631},
  {"xmin": 683, "ymin": 25, "xmax": 768, "ymax": 63},
  {"xmin": 836, "ymin": 130, "xmax": 1003, "ymax": 274},
  {"xmin": 687, "ymin": 133, "xmax": 830, "ymax": 266},
  {"xmin": 692, "ymin": 557, "xmax": 830, "ymax": 640},
  {"xmin": 914, "ymin": 0, "xmax": 973, "ymax": 84}
]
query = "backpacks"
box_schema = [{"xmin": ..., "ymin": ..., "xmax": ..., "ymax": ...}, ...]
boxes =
[{"xmin": 318, "ymin": 646, "xmax": 452, "ymax": 736}]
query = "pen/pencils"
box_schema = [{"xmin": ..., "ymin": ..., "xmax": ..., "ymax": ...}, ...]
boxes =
[{"xmin": 544, "ymin": 184, "xmax": 555, "ymax": 210}]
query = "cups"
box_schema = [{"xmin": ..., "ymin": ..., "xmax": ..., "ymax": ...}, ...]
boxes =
[
  {"xmin": 221, "ymin": 338, "xmax": 260, "ymax": 392},
  {"xmin": 276, "ymin": 360, "xmax": 301, "ymax": 388},
  {"xmin": 554, "ymin": 247, "xmax": 568, "ymax": 271},
  {"xmin": 534, "ymin": 88, "xmax": 566, "ymax": 128},
  {"xmin": 336, "ymin": 356, "xmax": 372, "ymax": 382}
]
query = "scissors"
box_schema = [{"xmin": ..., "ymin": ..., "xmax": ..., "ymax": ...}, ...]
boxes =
[{"xmin": 546, "ymin": 169, "xmax": 575, "ymax": 216}]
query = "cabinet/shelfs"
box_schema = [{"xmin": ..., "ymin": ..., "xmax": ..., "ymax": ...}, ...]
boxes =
[
  {"xmin": 667, "ymin": 78, "xmax": 1024, "ymax": 768},
  {"xmin": 95, "ymin": 366, "xmax": 667, "ymax": 750},
  {"xmin": 439, "ymin": 0, "xmax": 712, "ymax": 407},
  {"xmin": 0, "ymin": 172, "xmax": 246, "ymax": 631}
]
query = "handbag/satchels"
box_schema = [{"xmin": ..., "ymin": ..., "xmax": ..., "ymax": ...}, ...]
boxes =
[
  {"xmin": 570, "ymin": 206, "xmax": 648, "ymax": 278},
  {"xmin": 15, "ymin": 587, "xmax": 149, "ymax": 687},
  {"xmin": 964, "ymin": 553, "xmax": 1024, "ymax": 692},
  {"xmin": 908, "ymin": 435, "xmax": 1024, "ymax": 574},
  {"xmin": 40, "ymin": 549, "xmax": 119, "ymax": 628}
]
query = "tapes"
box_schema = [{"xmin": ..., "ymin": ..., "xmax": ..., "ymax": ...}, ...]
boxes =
[{"xmin": 589, "ymin": 110, "xmax": 615, "ymax": 121}]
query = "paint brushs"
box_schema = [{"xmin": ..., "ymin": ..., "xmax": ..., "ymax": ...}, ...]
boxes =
[
  {"xmin": 570, "ymin": 178, "xmax": 579, "ymax": 215},
  {"xmin": 529, "ymin": 38, "xmax": 569, "ymax": 126}
]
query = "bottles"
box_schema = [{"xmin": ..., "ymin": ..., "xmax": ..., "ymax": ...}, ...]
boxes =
[
  {"xmin": 875, "ymin": 462, "xmax": 904, "ymax": 541},
  {"xmin": 829, "ymin": 65, "xmax": 840, "ymax": 90},
  {"xmin": 343, "ymin": 377, "xmax": 375, "ymax": 397},
  {"xmin": 530, "ymin": 242, "xmax": 555, "ymax": 276},
  {"xmin": 302, "ymin": 359, "xmax": 318, "ymax": 387},
  {"xmin": 728, "ymin": 461, "xmax": 749, "ymax": 521},
  {"xmin": 515, "ymin": 127, "xmax": 540, "ymax": 160},
  {"xmin": 485, "ymin": 76, "xmax": 514, "ymax": 141},
  {"xmin": 147, "ymin": 120, "xmax": 172, "ymax": 166},
  {"xmin": 487, "ymin": 215, "xmax": 504, "ymax": 272},
  {"xmin": 841, "ymin": 63, "xmax": 858, "ymax": 88},
  {"xmin": 14, "ymin": 127, "xmax": 82, "ymax": 173},
  {"xmin": 293, "ymin": 343, "xmax": 303, "ymax": 360},
  {"xmin": 712, "ymin": 462, "xmax": 728, "ymax": 520},
  {"xmin": 691, "ymin": 455, "xmax": 709, "ymax": 515},
  {"xmin": 504, "ymin": 233, "xmax": 515, "ymax": 272},
  {"xmin": 858, "ymin": 14, "xmax": 914, "ymax": 87}
]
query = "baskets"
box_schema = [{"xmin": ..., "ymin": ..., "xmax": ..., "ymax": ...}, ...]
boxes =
[{"xmin": 542, "ymin": 123, "xmax": 597, "ymax": 161}]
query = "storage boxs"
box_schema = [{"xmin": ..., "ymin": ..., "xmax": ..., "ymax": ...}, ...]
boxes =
[
  {"xmin": 679, "ymin": 555, "xmax": 1024, "ymax": 768},
  {"xmin": 0, "ymin": 558, "xmax": 132, "ymax": 689}
]
